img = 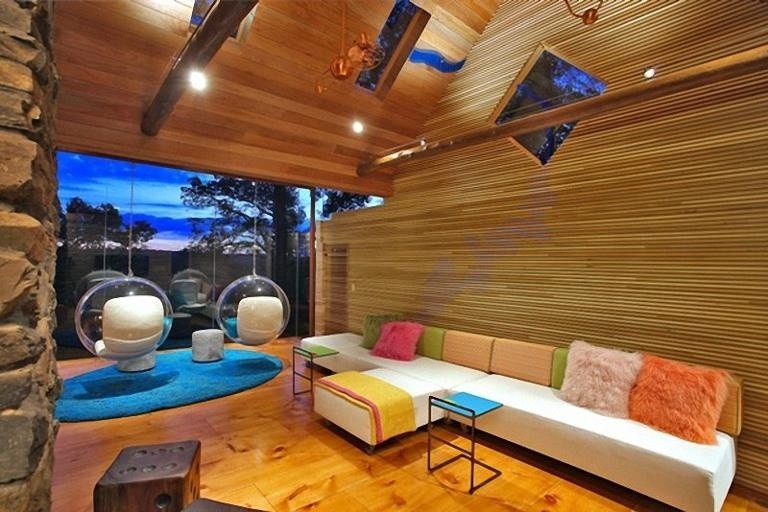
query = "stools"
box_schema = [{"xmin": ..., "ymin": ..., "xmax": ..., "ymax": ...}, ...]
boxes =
[
  {"xmin": 192, "ymin": 329, "xmax": 223, "ymax": 364},
  {"xmin": 166, "ymin": 310, "xmax": 192, "ymax": 349},
  {"xmin": 117, "ymin": 349, "xmax": 157, "ymax": 372}
]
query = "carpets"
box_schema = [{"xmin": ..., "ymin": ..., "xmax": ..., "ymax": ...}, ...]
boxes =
[{"xmin": 54, "ymin": 349, "xmax": 281, "ymax": 421}]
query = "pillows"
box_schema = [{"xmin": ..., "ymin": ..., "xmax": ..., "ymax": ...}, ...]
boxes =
[
  {"xmin": 629, "ymin": 352, "xmax": 728, "ymax": 445},
  {"xmin": 376, "ymin": 322, "xmax": 421, "ymax": 361},
  {"xmin": 560, "ymin": 339, "xmax": 643, "ymax": 418},
  {"xmin": 361, "ymin": 311, "xmax": 401, "ymax": 350}
]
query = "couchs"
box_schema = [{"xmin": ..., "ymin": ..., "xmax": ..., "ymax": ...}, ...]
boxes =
[
  {"xmin": 198, "ymin": 281, "xmax": 224, "ymax": 320},
  {"xmin": 300, "ymin": 321, "xmax": 745, "ymax": 511}
]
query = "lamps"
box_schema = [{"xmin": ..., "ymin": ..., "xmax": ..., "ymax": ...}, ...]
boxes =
[{"xmin": 642, "ymin": 66, "xmax": 657, "ymax": 81}]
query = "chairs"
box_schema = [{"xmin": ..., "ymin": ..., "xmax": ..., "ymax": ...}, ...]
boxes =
[
  {"xmin": 233, "ymin": 296, "xmax": 285, "ymax": 345},
  {"xmin": 86, "ymin": 278, "xmax": 114, "ymax": 308},
  {"xmin": 172, "ymin": 279, "xmax": 209, "ymax": 312},
  {"xmin": 93, "ymin": 295, "xmax": 166, "ymax": 359}
]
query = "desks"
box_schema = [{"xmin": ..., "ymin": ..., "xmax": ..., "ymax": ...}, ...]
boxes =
[
  {"xmin": 182, "ymin": 496, "xmax": 267, "ymax": 511},
  {"xmin": 94, "ymin": 440, "xmax": 201, "ymax": 512}
]
